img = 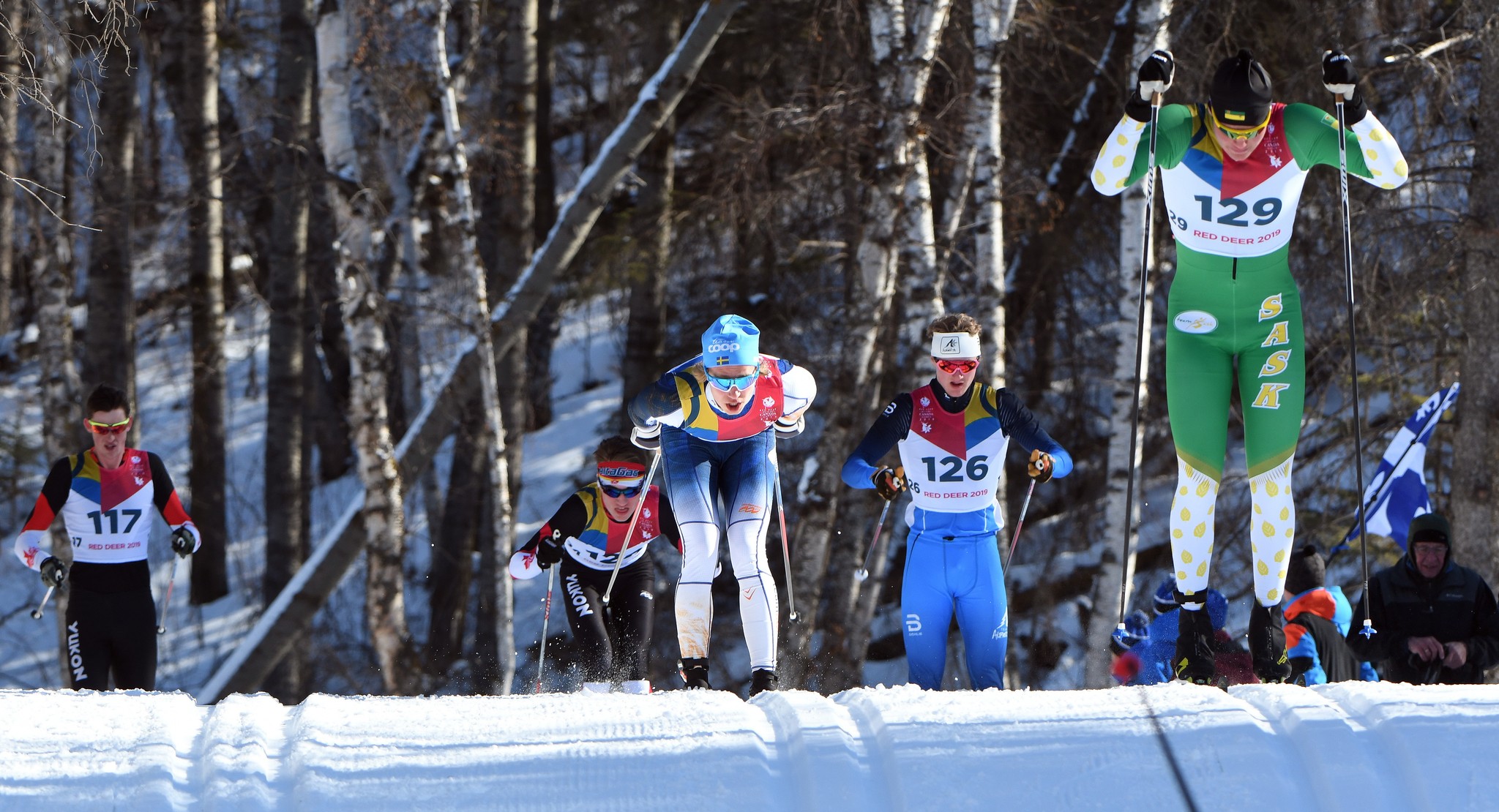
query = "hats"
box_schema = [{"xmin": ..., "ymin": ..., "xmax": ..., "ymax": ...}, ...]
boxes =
[
  {"xmin": 1208, "ymin": 47, "xmax": 1273, "ymax": 125},
  {"xmin": 1284, "ymin": 543, "xmax": 1326, "ymax": 594},
  {"xmin": 1408, "ymin": 513, "xmax": 1453, "ymax": 566},
  {"xmin": 1153, "ymin": 574, "xmax": 1179, "ymax": 618},
  {"xmin": 701, "ymin": 314, "xmax": 760, "ymax": 368},
  {"xmin": 1110, "ymin": 609, "xmax": 1151, "ymax": 658},
  {"xmin": 1206, "ymin": 585, "xmax": 1229, "ymax": 629}
]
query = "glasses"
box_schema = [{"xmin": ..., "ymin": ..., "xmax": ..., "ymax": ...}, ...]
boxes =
[
  {"xmin": 1414, "ymin": 543, "xmax": 1445, "ymax": 554},
  {"xmin": 702, "ymin": 364, "xmax": 760, "ymax": 393},
  {"xmin": 932, "ymin": 356, "xmax": 980, "ymax": 375},
  {"xmin": 88, "ymin": 418, "xmax": 130, "ymax": 435},
  {"xmin": 598, "ymin": 474, "xmax": 647, "ymax": 498},
  {"xmin": 1207, "ymin": 95, "xmax": 1272, "ymax": 140}
]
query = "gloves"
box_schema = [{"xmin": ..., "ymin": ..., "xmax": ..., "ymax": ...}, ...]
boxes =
[
  {"xmin": 630, "ymin": 423, "xmax": 662, "ymax": 454},
  {"xmin": 773, "ymin": 414, "xmax": 806, "ymax": 440},
  {"xmin": 1322, "ymin": 49, "xmax": 1369, "ymax": 127},
  {"xmin": 869, "ymin": 464, "xmax": 906, "ymax": 501},
  {"xmin": 1027, "ymin": 449, "xmax": 1056, "ymax": 483},
  {"xmin": 40, "ymin": 554, "xmax": 67, "ymax": 588},
  {"xmin": 1125, "ymin": 50, "xmax": 1176, "ymax": 123},
  {"xmin": 170, "ymin": 525, "xmax": 195, "ymax": 558},
  {"xmin": 533, "ymin": 534, "xmax": 565, "ymax": 569}
]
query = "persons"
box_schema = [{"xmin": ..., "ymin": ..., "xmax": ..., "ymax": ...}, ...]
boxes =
[
  {"xmin": 14, "ymin": 387, "xmax": 200, "ymax": 692},
  {"xmin": 627, "ymin": 315, "xmax": 816, "ymax": 698},
  {"xmin": 840, "ymin": 314, "xmax": 1074, "ymax": 692},
  {"xmin": 1111, "ymin": 543, "xmax": 1381, "ymax": 694},
  {"xmin": 508, "ymin": 436, "xmax": 722, "ymax": 692},
  {"xmin": 1091, "ymin": 48, "xmax": 1408, "ymax": 684},
  {"xmin": 1345, "ymin": 516, "xmax": 1499, "ymax": 684}
]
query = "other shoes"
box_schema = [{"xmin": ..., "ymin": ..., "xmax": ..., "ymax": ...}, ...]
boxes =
[
  {"xmin": 1174, "ymin": 603, "xmax": 1217, "ymax": 685},
  {"xmin": 1247, "ymin": 596, "xmax": 1291, "ymax": 679},
  {"xmin": 676, "ymin": 656, "xmax": 712, "ymax": 692},
  {"xmin": 748, "ymin": 666, "xmax": 781, "ymax": 698}
]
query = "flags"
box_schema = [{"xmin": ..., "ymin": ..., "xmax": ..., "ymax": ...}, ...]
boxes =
[{"xmin": 1346, "ymin": 383, "xmax": 1460, "ymax": 553}]
